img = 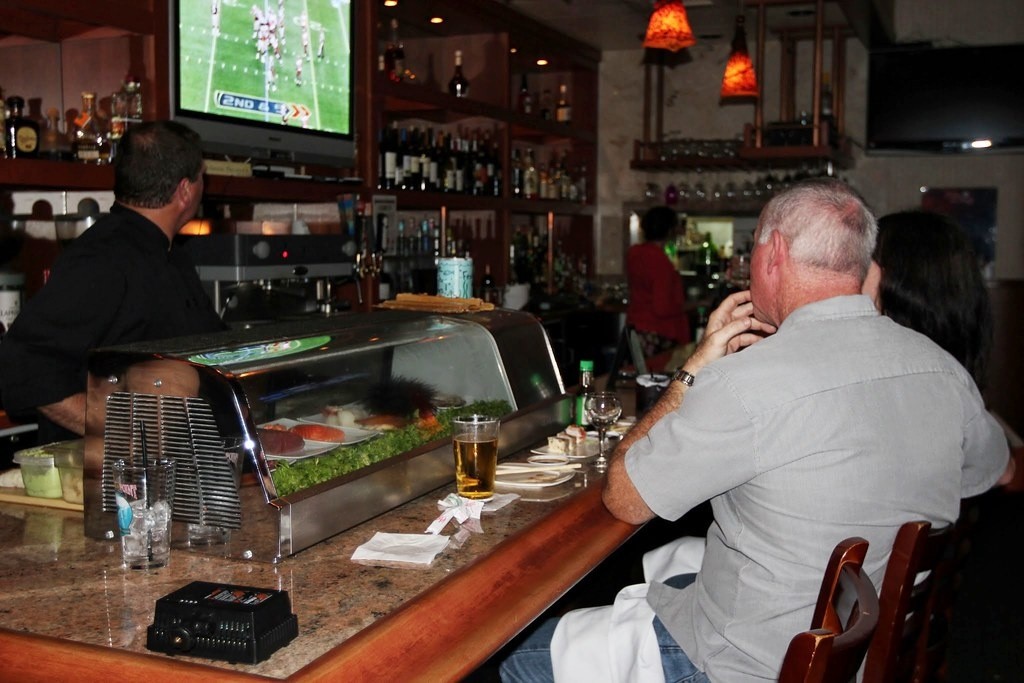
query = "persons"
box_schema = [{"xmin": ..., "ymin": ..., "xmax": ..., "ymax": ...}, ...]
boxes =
[
  {"xmin": 0, "ymin": 122, "xmax": 233, "ymax": 438},
  {"xmin": 615, "ymin": 206, "xmax": 712, "ymax": 365},
  {"xmin": 495, "ymin": 181, "xmax": 1024, "ymax": 683},
  {"xmin": 863, "ymin": 205, "xmax": 990, "ymax": 399},
  {"xmin": 208, "ymin": 0, "xmax": 327, "ymax": 126}
]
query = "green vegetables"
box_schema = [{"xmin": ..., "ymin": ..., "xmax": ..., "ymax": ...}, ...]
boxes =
[{"xmin": 270, "ymin": 398, "xmax": 512, "ymax": 497}]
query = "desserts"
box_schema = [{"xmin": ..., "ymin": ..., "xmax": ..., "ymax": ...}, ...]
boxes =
[{"xmin": 546, "ymin": 424, "xmax": 586, "ymax": 454}]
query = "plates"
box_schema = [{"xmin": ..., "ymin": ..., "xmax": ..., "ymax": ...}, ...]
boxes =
[
  {"xmin": 527, "ymin": 455, "xmax": 570, "ymax": 464},
  {"xmin": 255, "ymin": 394, "xmax": 467, "ymax": 473},
  {"xmin": 530, "ymin": 439, "xmax": 610, "ymax": 459},
  {"xmin": 494, "ymin": 463, "xmax": 575, "ymax": 487}
]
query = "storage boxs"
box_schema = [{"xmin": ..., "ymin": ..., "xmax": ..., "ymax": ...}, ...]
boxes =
[{"xmin": 12, "ymin": 437, "xmax": 84, "ymax": 504}]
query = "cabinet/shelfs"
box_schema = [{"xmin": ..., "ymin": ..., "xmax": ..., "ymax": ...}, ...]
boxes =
[{"xmin": 0, "ymin": 0, "xmax": 603, "ymax": 430}]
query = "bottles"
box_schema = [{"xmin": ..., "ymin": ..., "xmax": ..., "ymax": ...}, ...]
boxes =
[
  {"xmin": 379, "ymin": 18, "xmax": 589, "ymax": 295},
  {"xmin": 696, "ymin": 231, "xmax": 720, "ymax": 295},
  {"xmin": 0, "ymin": 81, "xmax": 143, "ymax": 165},
  {"xmin": 574, "ymin": 360, "xmax": 599, "ymax": 431}
]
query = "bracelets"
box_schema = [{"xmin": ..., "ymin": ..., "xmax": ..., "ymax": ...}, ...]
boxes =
[{"xmin": 672, "ymin": 369, "xmax": 694, "ymax": 387}]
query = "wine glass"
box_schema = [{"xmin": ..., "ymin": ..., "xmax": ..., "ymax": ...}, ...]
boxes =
[
  {"xmin": 584, "ymin": 392, "xmax": 621, "ymax": 466},
  {"xmin": 641, "ymin": 162, "xmax": 818, "ymax": 207}
]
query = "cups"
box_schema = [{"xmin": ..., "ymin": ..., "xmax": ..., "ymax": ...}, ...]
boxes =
[
  {"xmin": 436, "ymin": 258, "xmax": 473, "ymax": 299},
  {"xmin": 112, "ymin": 455, "xmax": 175, "ymax": 572},
  {"xmin": 730, "ymin": 256, "xmax": 751, "ymax": 279},
  {"xmin": 452, "ymin": 415, "xmax": 500, "ymax": 500},
  {"xmin": 635, "ymin": 374, "xmax": 670, "ymax": 420}
]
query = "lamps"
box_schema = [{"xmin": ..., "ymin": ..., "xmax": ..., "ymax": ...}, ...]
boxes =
[
  {"xmin": 641, "ymin": 0, "xmax": 697, "ymax": 51},
  {"xmin": 718, "ymin": 0, "xmax": 760, "ymax": 108}
]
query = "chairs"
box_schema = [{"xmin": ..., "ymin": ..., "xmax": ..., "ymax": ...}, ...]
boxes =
[
  {"xmin": 777, "ymin": 537, "xmax": 879, "ymax": 683},
  {"xmin": 863, "ymin": 503, "xmax": 976, "ymax": 683}
]
